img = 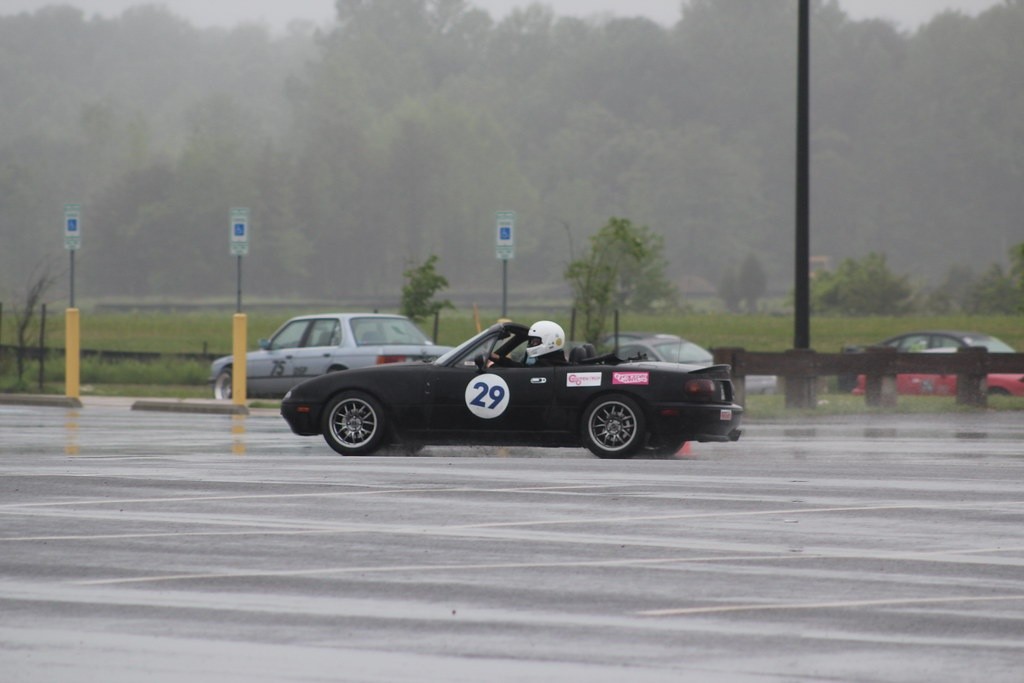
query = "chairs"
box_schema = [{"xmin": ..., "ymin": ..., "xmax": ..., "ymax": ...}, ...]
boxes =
[
  {"xmin": 568, "ymin": 343, "xmax": 596, "ymax": 366},
  {"xmin": 319, "ymin": 331, "xmax": 332, "ymax": 345}
]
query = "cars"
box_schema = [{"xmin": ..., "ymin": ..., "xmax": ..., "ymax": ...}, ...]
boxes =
[
  {"xmin": 207, "ymin": 313, "xmax": 462, "ymax": 403},
  {"xmin": 850, "ymin": 329, "xmax": 1024, "ymax": 401},
  {"xmin": 602, "ymin": 332, "xmax": 715, "ymax": 369}
]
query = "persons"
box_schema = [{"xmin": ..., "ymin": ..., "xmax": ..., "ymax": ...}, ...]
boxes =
[{"xmin": 481, "ymin": 321, "xmax": 569, "ymax": 369}]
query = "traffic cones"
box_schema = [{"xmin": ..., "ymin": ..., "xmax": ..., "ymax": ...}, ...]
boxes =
[{"xmin": 672, "ymin": 440, "xmax": 699, "ymax": 461}]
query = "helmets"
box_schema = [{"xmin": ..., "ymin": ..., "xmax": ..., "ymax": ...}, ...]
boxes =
[{"xmin": 526, "ymin": 320, "xmax": 565, "ymax": 357}]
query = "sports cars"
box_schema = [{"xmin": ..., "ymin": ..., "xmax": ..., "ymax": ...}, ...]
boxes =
[{"xmin": 280, "ymin": 321, "xmax": 742, "ymax": 462}]
